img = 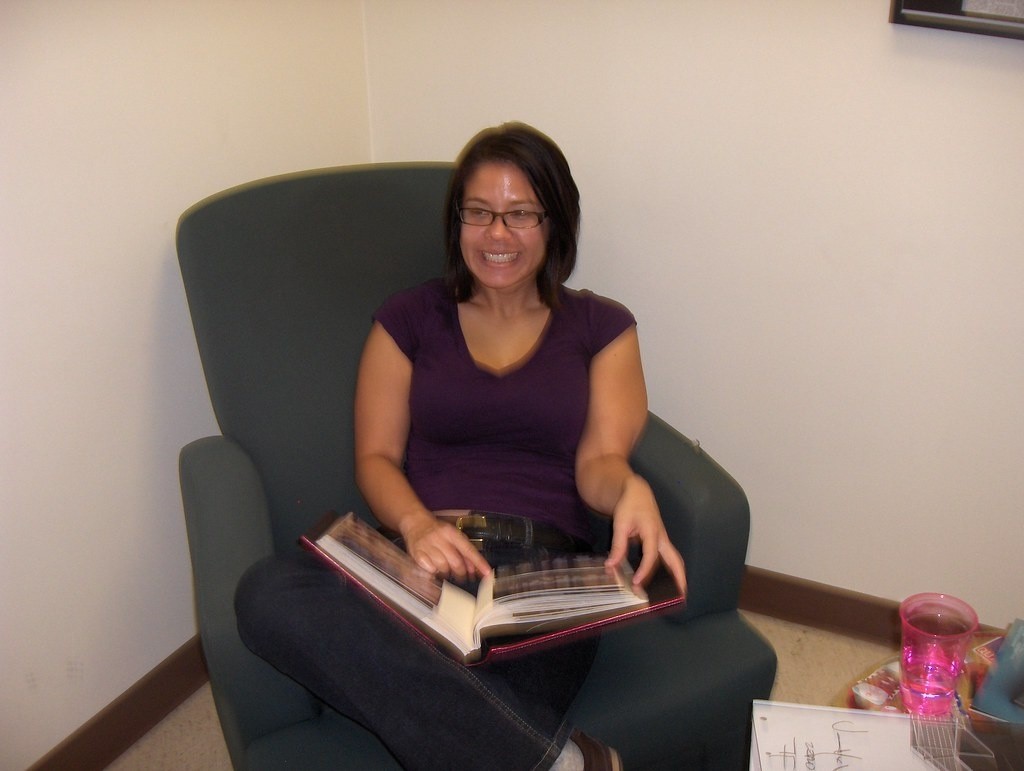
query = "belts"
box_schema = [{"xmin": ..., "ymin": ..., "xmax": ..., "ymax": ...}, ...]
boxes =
[{"xmin": 441, "ymin": 515, "xmax": 595, "ymax": 556}]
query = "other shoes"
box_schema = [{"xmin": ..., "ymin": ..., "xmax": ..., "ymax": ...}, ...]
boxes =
[{"xmin": 566, "ymin": 727, "xmax": 622, "ymax": 771}]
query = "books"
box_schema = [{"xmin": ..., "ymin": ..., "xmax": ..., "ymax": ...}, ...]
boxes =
[{"xmin": 301, "ymin": 507, "xmax": 687, "ymax": 668}]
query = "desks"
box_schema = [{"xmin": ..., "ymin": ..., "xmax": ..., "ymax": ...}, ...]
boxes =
[{"xmin": 828, "ymin": 628, "xmax": 1024, "ymax": 771}]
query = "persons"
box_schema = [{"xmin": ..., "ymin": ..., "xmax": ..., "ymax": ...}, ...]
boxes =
[{"xmin": 235, "ymin": 122, "xmax": 687, "ymax": 771}]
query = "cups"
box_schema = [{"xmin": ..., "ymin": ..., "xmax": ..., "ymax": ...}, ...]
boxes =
[{"xmin": 898, "ymin": 592, "xmax": 978, "ymax": 716}]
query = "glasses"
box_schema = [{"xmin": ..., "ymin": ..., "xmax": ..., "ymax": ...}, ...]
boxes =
[{"xmin": 454, "ymin": 200, "xmax": 553, "ymax": 230}]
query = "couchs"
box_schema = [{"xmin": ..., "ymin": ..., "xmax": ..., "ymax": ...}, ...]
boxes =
[{"xmin": 176, "ymin": 160, "xmax": 779, "ymax": 771}]
